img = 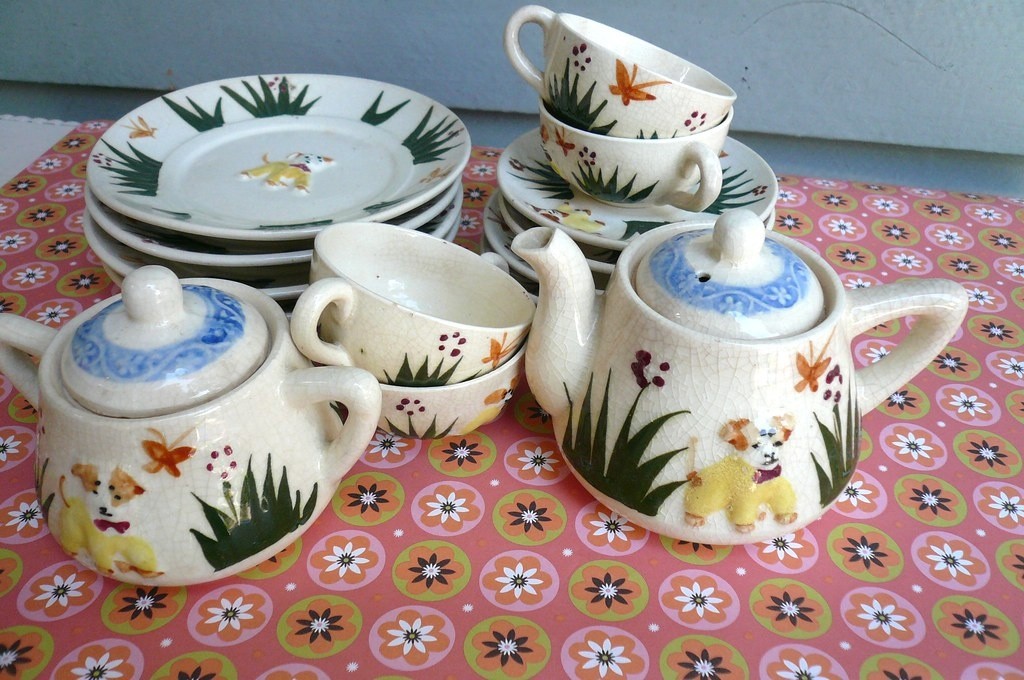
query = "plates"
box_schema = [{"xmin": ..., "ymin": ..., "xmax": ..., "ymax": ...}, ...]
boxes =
[
  {"xmin": 84, "ymin": 73, "xmax": 472, "ymax": 320},
  {"xmin": 481, "ymin": 127, "xmax": 779, "ymax": 313}
]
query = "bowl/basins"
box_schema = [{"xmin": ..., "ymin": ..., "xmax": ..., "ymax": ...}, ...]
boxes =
[
  {"xmin": 324, "ymin": 292, "xmax": 539, "ymax": 440},
  {"xmin": 539, "ymin": 97, "xmax": 733, "ymax": 213},
  {"xmin": 292, "ymin": 221, "xmax": 536, "ymax": 388},
  {"xmin": 505, "ymin": 6, "xmax": 738, "ymax": 138}
]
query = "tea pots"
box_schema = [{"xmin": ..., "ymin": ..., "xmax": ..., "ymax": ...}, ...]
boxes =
[{"xmin": 512, "ymin": 209, "xmax": 967, "ymax": 546}]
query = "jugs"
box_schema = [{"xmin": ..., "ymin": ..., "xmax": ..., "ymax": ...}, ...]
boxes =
[{"xmin": 0, "ymin": 266, "xmax": 381, "ymax": 588}]
